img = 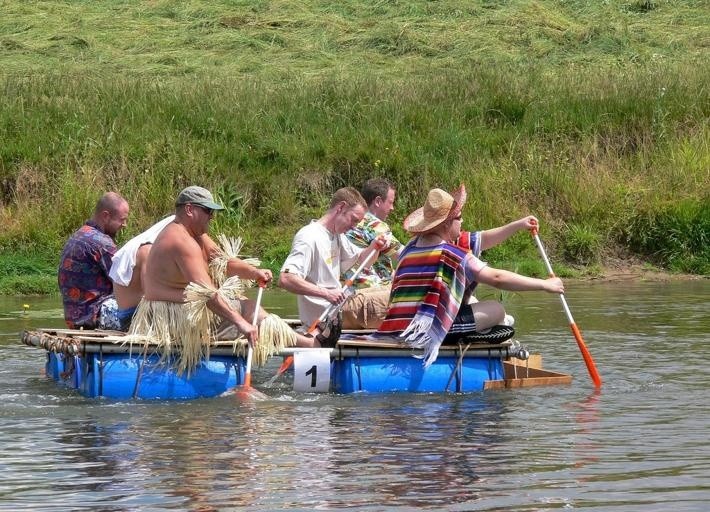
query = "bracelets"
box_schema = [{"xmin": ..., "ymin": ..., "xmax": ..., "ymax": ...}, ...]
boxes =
[{"xmin": 249, "ymin": 267, "xmax": 256, "ymax": 280}]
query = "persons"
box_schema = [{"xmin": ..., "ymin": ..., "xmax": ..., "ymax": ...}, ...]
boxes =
[
  {"xmin": 58, "ymin": 191, "xmax": 129, "ymax": 330},
  {"xmin": 278, "ymin": 185, "xmax": 393, "ymax": 330},
  {"xmin": 116, "ymin": 186, "xmax": 341, "ymax": 376},
  {"xmin": 355, "ymin": 183, "xmax": 565, "ymax": 369},
  {"xmin": 108, "ymin": 214, "xmax": 176, "ymax": 330},
  {"xmin": 340, "ymin": 178, "xmax": 404, "ymax": 289}
]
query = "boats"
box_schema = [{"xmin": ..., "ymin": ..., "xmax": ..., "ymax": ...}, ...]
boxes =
[{"xmin": 23, "ymin": 321, "xmax": 571, "ymax": 400}]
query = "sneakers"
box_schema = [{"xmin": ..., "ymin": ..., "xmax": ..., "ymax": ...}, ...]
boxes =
[{"xmin": 463, "ymin": 325, "xmax": 515, "ymax": 344}]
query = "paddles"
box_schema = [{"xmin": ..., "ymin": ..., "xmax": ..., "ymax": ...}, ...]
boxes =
[
  {"xmin": 261, "ymin": 235, "xmax": 386, "ymax": 389},
  {"xmin": 530, "ymin": 220, "xmax": 601, "ymax": 386}
]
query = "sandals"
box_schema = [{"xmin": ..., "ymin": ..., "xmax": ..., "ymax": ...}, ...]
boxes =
[
  {"xmin": 316, "ymin": 312, "xmax": 343, "ymax": 348},
  {"xmin": 304, "ymin": 322, "xmax": 327, "ymax": 339}
]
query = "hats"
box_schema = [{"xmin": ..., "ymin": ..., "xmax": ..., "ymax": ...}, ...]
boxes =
[
  {"xmin": 403, "ymin": 183, "xmax": 466, "ymax": 233},
  {"xmin": 176, "ymin": 186, "xmax": 226, "ymax": 211}
]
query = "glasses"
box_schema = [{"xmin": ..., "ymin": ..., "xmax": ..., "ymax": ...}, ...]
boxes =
[
  {"xmin": 453, "ymin": 215, "xmax": 462, "ymax": 221},
  {"xmin": 191, "ymin": 204, "xmax": 214, "ymax": 215}
]
